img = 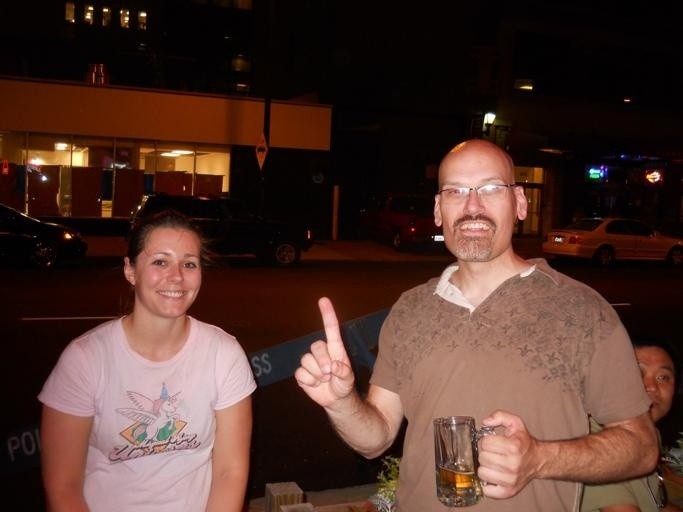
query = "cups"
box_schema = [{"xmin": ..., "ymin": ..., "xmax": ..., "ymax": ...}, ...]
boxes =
[{"xmin": 433, "ymin": 415, "xmax": 500, "ymax": 508}]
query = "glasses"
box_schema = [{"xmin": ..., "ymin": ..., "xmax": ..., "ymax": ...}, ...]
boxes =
[{"xmin": 439, "ymin": 185, "xmax": 515, "ymax": 201}]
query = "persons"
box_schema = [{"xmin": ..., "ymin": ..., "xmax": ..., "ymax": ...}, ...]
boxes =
[
  {"xmin": 294, "ymin": 139, "xmax": 660, "ymax": 512},
  {"xmin": 37, "ymin": 208, "xmax": 257, "ymax": 512},
  {"xmin": 579, "ymin": 330, "xmax": 683, "ymax": 512}
]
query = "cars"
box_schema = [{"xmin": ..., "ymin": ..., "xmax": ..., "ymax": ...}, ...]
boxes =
[
  {"xmin": 541, "ymin": 217, "xmax": 682, "ymax": 268},
  {"xmin": 360, "ymin": 187, "xmax": 442, "ymax": 250},
  {"xmin": 0, "ymin": 200, "xmax": 89, "ymax": 269}
]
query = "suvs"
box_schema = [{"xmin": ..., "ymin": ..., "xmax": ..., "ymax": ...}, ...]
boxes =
[{"xmin": 131, "ymin": 193, "xmax": 312, "ymax": 270}]
